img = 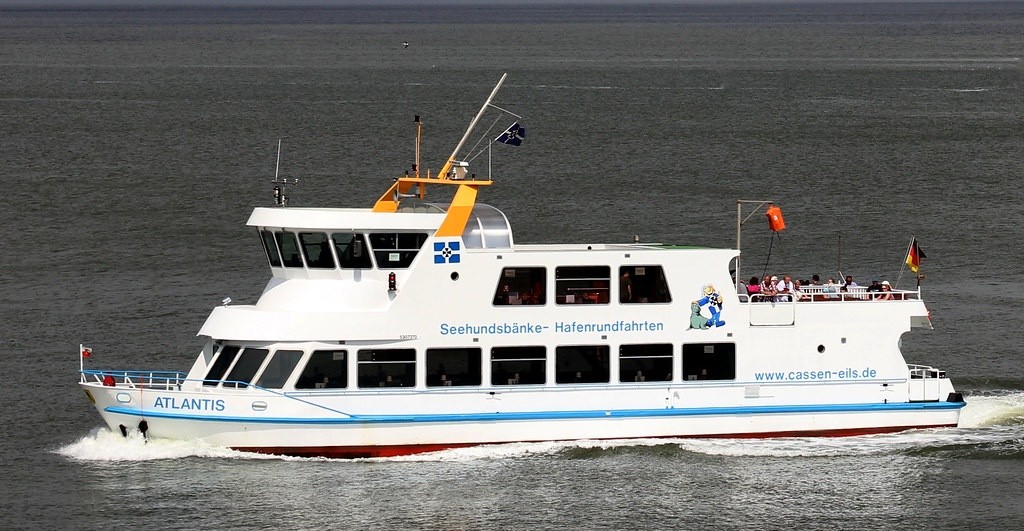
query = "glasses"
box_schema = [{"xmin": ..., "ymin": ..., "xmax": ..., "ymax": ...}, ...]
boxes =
[{"xmin": 882, "ymin": 288, "xmax": 887, "ymax": 289}]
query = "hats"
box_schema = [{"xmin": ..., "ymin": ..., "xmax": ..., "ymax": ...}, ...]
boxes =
[{"xmin": 770, "ymin": 276, "xmax": 778, "ymax": 281}]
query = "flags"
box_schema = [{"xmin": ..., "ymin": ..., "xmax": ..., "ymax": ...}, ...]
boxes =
[
  {"xmin": 905, "ymin": 237, "xmax": 927, "ymax": 274},
  {"xmin": 496, "ymin": 123, "xmax": 525, "ymax": 146}
]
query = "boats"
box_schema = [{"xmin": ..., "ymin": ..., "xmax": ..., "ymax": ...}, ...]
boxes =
[{"xmin": 80, "ymin": 72, "xmax": 969, "ymax": 457}]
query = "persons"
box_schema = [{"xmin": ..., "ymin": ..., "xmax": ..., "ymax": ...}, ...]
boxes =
[
  {"xmin": 828, "ymin": 280, "xmax": 835, "ymax": 293},
  {"xmin": 522, "ymin": 292, "xmax": 534, "ymax": 305},
  {"xmin": 748, "ymin": 275, "xmax": 807, "ymax": 302},
  {"xmin": 811, "ymin": 274, "xmax": 823, "ymax": 285},
  {"xmin": 581, "ymin": 292, "xmax": 595, "ymax": 304},
  {"xmin": 622, "ymin": 273, "xmax": 632, "ymax": 303},
  {"xmin": 844, "ymin": 275, "xmax": 852, "ymax": 292},
  {"xmin": 866, "ymin": 281, "xmax": 895, "ymax": 300}
]
